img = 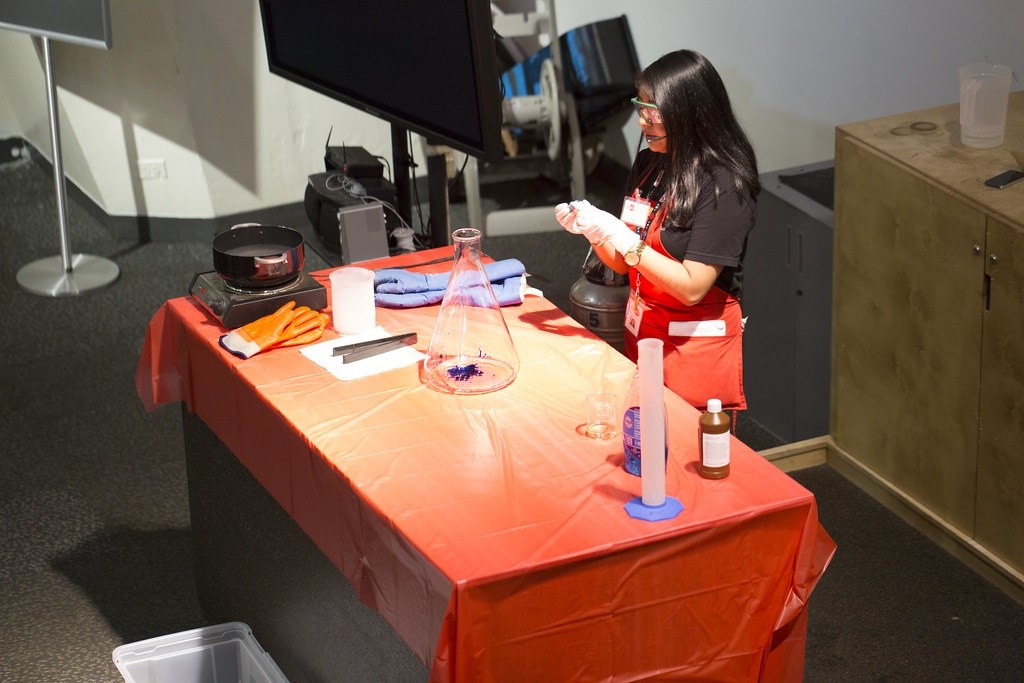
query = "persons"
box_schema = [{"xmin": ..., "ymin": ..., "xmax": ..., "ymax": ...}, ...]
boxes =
[{"xmin": 554, "ymin": 49, "xmax": 760, "ymax": 414}]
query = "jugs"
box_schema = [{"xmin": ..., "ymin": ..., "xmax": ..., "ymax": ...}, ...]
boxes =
[{"xmin": 959, "ymin": 64, "xmax": 1013, "ymax": 149}]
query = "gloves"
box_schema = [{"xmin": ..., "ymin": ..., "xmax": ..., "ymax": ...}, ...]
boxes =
[
  {"xmin": 218, "ymin": 301, "xmax": 331, "ymax": 359},
  {"xmin": 555, "ymin": 199, "xmax": 641, "ymax": 255},
  {"xmin": 373, "ymin": 258, "xmax": 528, "ymax": 307}
]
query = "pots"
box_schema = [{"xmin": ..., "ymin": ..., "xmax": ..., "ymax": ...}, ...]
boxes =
[{"xmin": 212, "ymin": 223, "xmax": 305, "ymax": 288}]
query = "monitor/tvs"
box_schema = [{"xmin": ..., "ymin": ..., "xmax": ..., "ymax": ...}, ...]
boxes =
[{"xmin": 259, "ymin": 0, "xmax": 507, "ymax": 164}]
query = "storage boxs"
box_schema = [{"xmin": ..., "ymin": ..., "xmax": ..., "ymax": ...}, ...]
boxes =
[{"xmin": 113, "ymin": 621, "xmax": 289, "ymax": 682}]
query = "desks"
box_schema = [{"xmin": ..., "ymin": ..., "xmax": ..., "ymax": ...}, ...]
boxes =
[{"xmin": 135, "ymin": 243, "xmax": 838, "ymax": 683}]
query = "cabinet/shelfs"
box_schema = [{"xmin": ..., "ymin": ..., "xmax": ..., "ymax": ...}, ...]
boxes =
[
  {"xmin": 825, "ymin": 91, "xmax": 1023, "ymax": 606},
  {"xmin": 731, "ymin": 157, "xmax": 836, "ymax": 444}
]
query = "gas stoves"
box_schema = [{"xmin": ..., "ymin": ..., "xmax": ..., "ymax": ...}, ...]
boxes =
[{"xmin": 188, "ymin": 269, "xmax": 328, "ymax": 328}]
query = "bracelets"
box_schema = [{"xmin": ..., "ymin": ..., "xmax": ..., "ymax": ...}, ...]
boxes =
[{"xmin": 592, "ymin": 241, "xmax": 605, "ymax": 246}]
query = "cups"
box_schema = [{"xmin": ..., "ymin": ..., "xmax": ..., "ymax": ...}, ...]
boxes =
[
  {"xmin": 585, "ymin": 392, "xmax": 618, "ymax": 439},
  {"xmin": 329, "ymin": 267, "xmax": 375, "ymax": 335}
]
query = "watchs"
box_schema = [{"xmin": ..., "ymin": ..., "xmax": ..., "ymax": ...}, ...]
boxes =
[{"xmin": 624, "ymin": 241, "xmax": 646, "ymax": 266}]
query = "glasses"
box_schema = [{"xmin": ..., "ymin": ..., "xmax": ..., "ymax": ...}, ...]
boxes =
[{"xmin": 629, "ymin": 95, "xmax": 664, "ymax": 124}]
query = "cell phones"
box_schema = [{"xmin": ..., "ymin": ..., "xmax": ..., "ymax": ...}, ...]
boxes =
[{"xmin": 985, "ymin": 170, "xmax": 1024, "ymax": 190}]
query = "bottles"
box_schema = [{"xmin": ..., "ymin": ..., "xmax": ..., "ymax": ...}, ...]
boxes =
[
  {"xmin": 620, "ymin": 358, "xmax": 668, "ymax": 476},
  {"xmin": 698, "ymin": 397, "xmax": 731, "ymax": 480},
  {"xmin": 425, "ymin": 228, "xmax": 521, "ymax": 396}
]
